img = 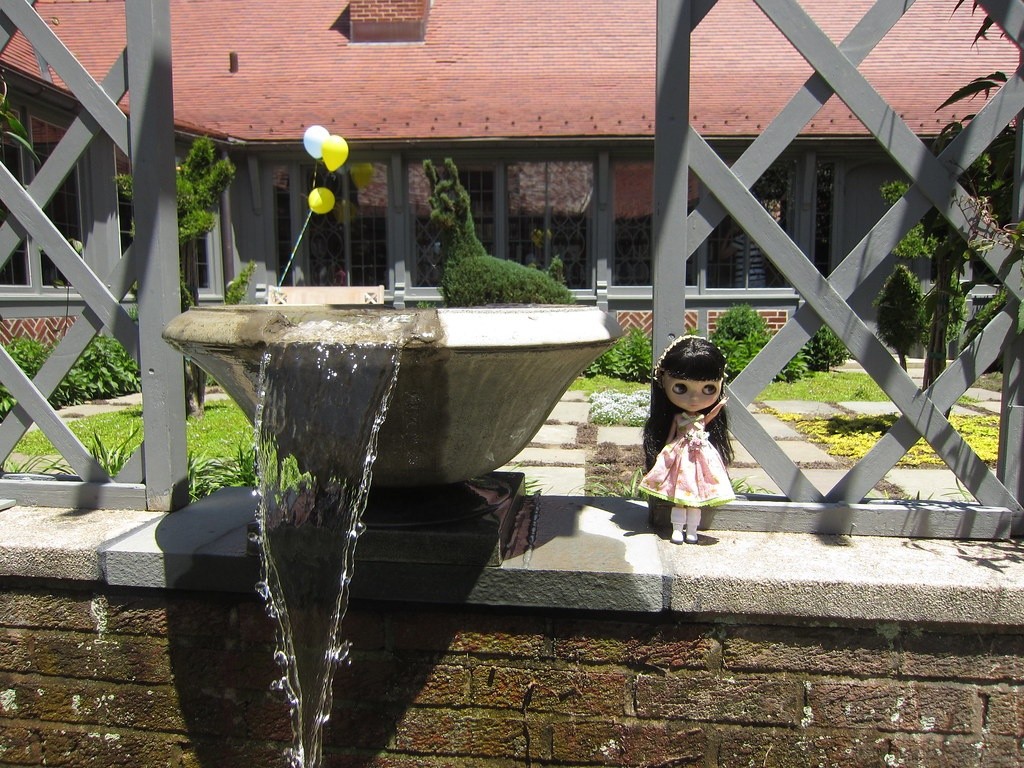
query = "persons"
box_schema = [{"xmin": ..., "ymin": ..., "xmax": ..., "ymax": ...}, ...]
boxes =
[{"xmin": 641, "ymin": 332, "xmax": 736, "ymax": 544}]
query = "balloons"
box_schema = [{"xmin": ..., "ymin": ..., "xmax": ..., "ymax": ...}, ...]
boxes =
[
  {"xmin": 303, "ymin": 125, "xmax": 331, "ymax": 160},
  {"xmin": 321, "ymin": 135, "xmax": 349, "ymax": 172},
  {"xmin": 308, "ymin": 187, "xmax": 335, "ymax": 214}
]
